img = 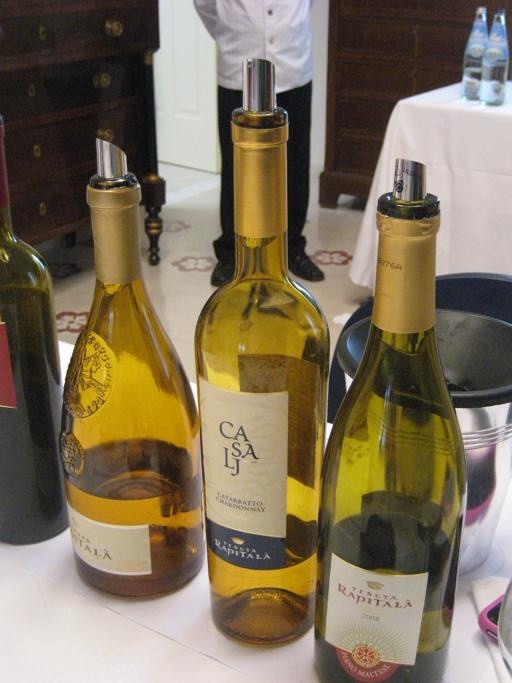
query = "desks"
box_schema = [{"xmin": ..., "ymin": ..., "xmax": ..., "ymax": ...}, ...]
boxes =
[
  {"xmin": 382, "ymin": 78, "xmax": 512, "ymax": 279},
  {"xmin": 3, "ymin": 342, "xmax": 512, "ymax": 683}
]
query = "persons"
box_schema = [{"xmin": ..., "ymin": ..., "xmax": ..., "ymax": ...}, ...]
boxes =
[{"xmin": 194, "ymin": 0, "xmax": 325, "ymax": 285}]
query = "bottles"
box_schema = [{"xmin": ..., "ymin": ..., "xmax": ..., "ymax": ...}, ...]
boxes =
[
  {"xmin": 462, "ymin": 8, "xmax": 509, "ymax": 107},
  {"xmin": 1, "ymin": 110, "xmax": 69, "ymax": 549},
  {"xmin": 56, "ymin": 137, "xmax": 208, "ymax": 600},
  {"xmin": 314, "ymin": 156, "xmax": 470, "ymax": 682},
  {"xmin": 195, "ymin": 58, "xmax": 332, "ymax": 646}
]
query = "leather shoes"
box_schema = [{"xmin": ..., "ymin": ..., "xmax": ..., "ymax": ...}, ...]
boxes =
[
  {"xmin": 288, "ymin": 243, "xmax": 324, "ymax": 282},
  {"xmin": 210, "ymin": 254, "xmax": 237, "ymax": 286}
]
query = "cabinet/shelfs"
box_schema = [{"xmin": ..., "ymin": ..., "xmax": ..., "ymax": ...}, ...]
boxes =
[
  {"xmin": 0, "ymin": 1, "xmax": 162, "ymax": 271},
  {"xmin": 316, "ymin": 1, "xmax": 510, "ymax": 207}
]
